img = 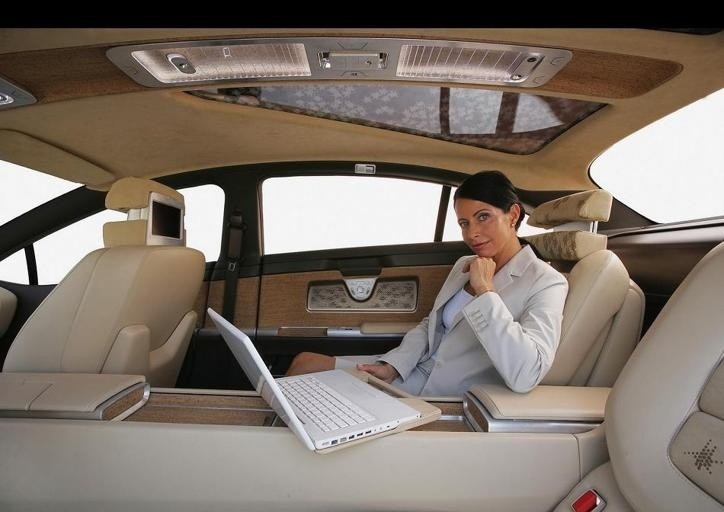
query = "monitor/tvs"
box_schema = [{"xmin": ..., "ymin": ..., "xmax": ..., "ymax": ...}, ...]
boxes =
[{"xmin": 150, "ymin": 200, "xmax": 182, "ymax": 240}]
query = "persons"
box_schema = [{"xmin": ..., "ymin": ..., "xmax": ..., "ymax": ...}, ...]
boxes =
[{"xmin": 282, "ymin": 170, "xmax": 569, "ymax": 398}]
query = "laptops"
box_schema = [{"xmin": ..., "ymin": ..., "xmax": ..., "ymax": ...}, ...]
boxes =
[{"xmin": 205, "ymin": 307, "xmax": 442, "ymax": 456}]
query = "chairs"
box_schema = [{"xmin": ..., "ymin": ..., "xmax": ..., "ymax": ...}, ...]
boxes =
[
  {"xmin": 520, "ymin": 189, "xmax": 649, "ymax": 384},
  {"xmin": 550, "ymin": 239, "xmax": 724, "ymax": 512},
  {"xmin": 1, "ymin": 175, "xmax": 211, "ymax": 388}
]
